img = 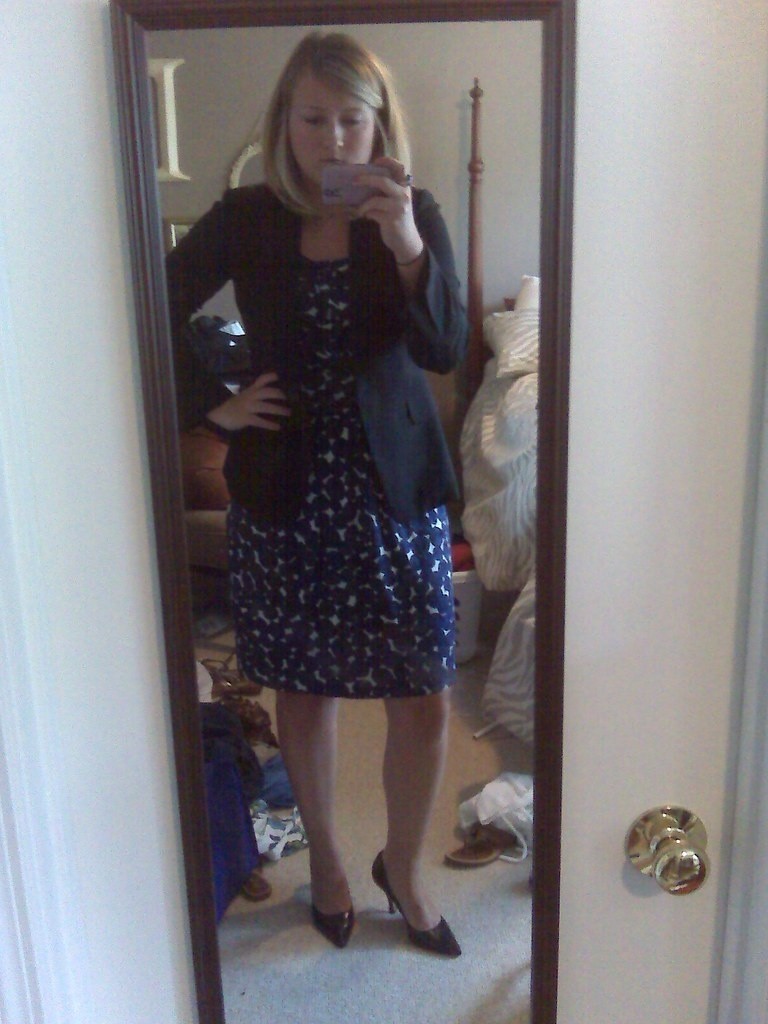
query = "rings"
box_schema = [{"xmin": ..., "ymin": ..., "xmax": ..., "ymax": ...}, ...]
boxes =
[{"xmin": 399, "ymin": 174, "xmax": 413, "ymax": 189}]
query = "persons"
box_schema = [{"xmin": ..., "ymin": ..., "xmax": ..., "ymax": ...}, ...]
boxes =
[{"xmin": 167, "ymin": 28, "xmax": 466, "ymax": 960}]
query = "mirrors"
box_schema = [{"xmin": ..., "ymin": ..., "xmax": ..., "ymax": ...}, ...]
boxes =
[{"xmin": 109, "ymin": 0, "xmax": 576, "ymax": 1024}]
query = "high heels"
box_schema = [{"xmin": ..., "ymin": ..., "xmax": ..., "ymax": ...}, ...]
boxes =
[
  {"xmin": 311, "ymin": 901, "xmax": 354, "ymax": 949},
  {"xmin": 371, "ymin": 849, "xmax": 464, "ymax": 957}
]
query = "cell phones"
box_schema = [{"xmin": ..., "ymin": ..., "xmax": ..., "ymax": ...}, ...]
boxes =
[{"xmin": 322, "ymin": 162, "xmax": 393, "ymax": 206}]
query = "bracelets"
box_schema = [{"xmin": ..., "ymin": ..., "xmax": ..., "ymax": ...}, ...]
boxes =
[{"xmin": 393, "ymin": 243, "xmax": 425, "ymax": 267}]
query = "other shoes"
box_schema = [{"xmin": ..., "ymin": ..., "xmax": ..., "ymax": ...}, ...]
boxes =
[{"xmin": 200, "ymin": 649, "xmax": 261, "ymax": 697}]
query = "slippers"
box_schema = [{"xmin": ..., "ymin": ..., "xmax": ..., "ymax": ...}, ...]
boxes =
[
  {"xmin": 240, "ymin": 872, "xmax": 272, "ymax": 902},
  {"xmin": 443, "ymin": 826, "xmax": 512, "ymax": 868}
]
